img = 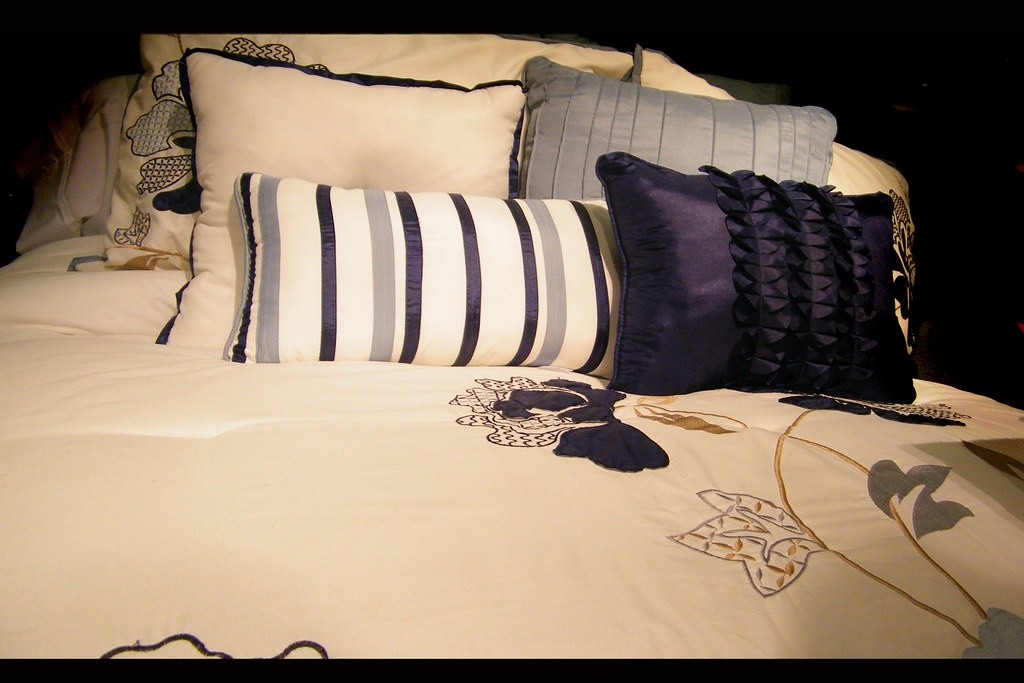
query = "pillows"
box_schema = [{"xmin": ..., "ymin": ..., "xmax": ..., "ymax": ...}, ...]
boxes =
[
  {"xmin": 594, "ymin": 152, "xmax": 917, "ymax": 407},
  {"xmin": 66, "ymin": 33, "xmax": 635, "ymax": 271},
  {"xmin": 632, "ymin": 43, "xmax": 915, "ymax": 354},
  {"xmin": 219, "ymin": 173, "xmax": 624, "ymax": 381},
  {"xmin": 154, "ymin": 45, "xmax": 528, "ymax": 358},
  {"xmin": 519, "ymin": 55, "xmax": 838, "ymax": 200}
]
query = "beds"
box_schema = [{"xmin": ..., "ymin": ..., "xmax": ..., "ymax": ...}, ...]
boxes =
[{"xmin": 1, "ymin": 229, "xmax": 1024, "ymax": 660}]
